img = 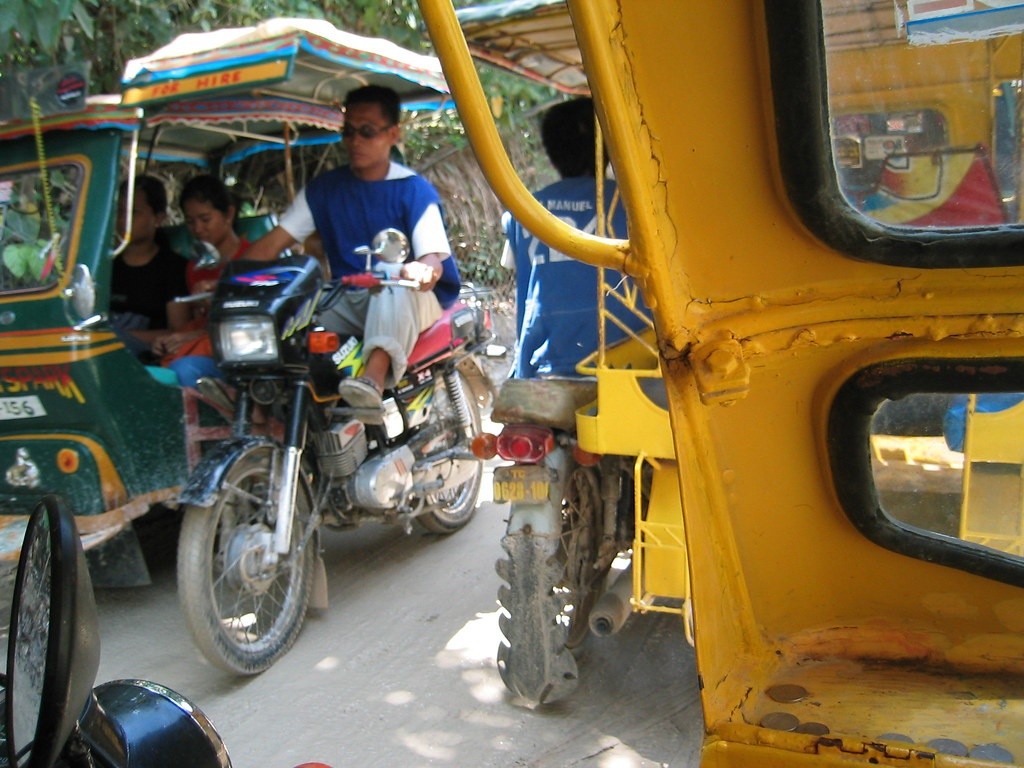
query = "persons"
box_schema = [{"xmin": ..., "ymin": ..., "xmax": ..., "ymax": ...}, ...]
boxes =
[
  {"xmin": 151, "ymin": 174, "xmax": 262, "ymax": 419},
  {"xmin": 109, "ymin": 174, "xmax": 191, "ymax": 366},
  {"xmin": 499, "ymin": 95, "xmax": 670, "ymax": 389},
  {"xmin": 239, "ymin": 83, "xmax": 462, "ymax": 426}
]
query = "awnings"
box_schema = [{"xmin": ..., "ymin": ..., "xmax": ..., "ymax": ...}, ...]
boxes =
[{"xmin": 418, "ymin": 1, "xmax": 599, "ymax": 95}]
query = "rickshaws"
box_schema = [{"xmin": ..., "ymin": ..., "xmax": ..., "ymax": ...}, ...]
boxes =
[
  {"xmin": 0, "ymin": 18, "xmax": 506, "ymax": 676},
  {"xmin": 418, "ymin": 0, "xmax": 1022, "ymax": 768}
]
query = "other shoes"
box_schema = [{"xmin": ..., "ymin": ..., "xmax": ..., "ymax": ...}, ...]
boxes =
[{"xmin": 338, "ymin": 377, "xmax": 384, "ymax": 426}]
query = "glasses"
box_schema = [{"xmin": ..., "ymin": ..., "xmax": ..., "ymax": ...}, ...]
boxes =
[{"xmin": 343, "ymin": 123, "xmax": 394, "ymax": 138}]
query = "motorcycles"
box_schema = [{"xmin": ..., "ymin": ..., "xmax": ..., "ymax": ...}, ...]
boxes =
[{"xmin": 0, "ymin": 496, "xmax": 231, "ymax": 768}]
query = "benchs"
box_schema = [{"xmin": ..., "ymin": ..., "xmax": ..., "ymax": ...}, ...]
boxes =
[{"xmin": 156, "ymin": 211, "xmax": 294, "ymax": 263}]
